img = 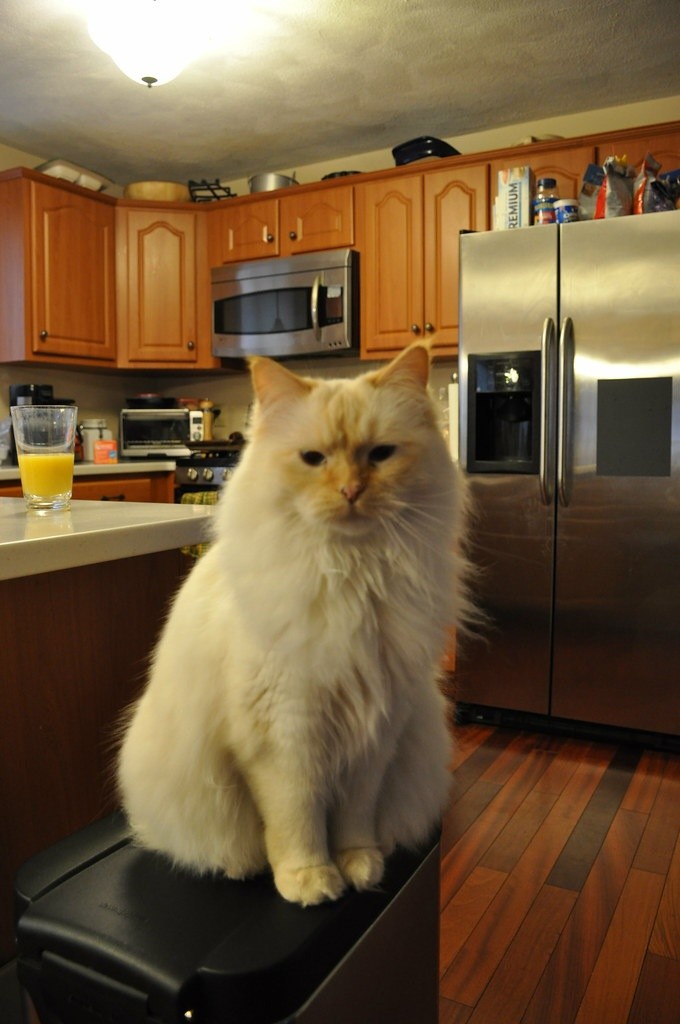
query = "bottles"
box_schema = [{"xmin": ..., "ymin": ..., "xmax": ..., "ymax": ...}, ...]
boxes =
[{"xmin": 535, "ymin": 178, "xmax": 559, "ymax": 226}]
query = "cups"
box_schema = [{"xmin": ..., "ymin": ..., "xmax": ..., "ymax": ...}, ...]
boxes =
[{"xmin": 9, "ymin": 406, "xmax": 78, "ymax": 510}]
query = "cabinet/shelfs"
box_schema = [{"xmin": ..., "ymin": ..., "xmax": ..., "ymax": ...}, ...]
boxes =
[
  {"xmin": 0, "ymin": 166, "xmax": 117, "ymax": 374},
  {"xmin": 114, "ymin": 199, "xmax": 248, "ymax": 377},
  {"xmin": 0, "ymin": 471, "xmax": 176, "ymax": 504},
  {"xmin": 209, "ymin": 172, "xmax": 359, "ymax": 269},
  {"xmin": 359, "ymin": 150, "xmax": 490, "ymax": 363},
  {"xmin": 490, "ymin": 119, "xmax": 680, "ymax": 230}
]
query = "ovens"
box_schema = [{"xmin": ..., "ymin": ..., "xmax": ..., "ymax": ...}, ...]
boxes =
[{"xmin": 211, "ymin": 249, "xmax": 360, "ymax": 360}]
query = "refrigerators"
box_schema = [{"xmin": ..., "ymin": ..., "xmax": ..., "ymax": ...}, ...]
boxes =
[{"xmin": 453, "ymin": 211, "xmax": 680, "ymax": 748}]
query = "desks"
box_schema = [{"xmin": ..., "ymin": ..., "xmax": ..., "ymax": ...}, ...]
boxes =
[{"xmin": 0, "ymin": 498, "xmax": 221, "ymax": 972}]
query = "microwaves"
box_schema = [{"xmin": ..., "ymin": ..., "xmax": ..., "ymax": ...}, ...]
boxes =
[{"xmin": 119, "ymin": 408, "xmax": 204, "ymax": 456}]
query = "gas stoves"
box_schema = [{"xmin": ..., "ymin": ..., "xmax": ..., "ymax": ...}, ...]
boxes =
[{"xmin": 173, "ymin": 449, "xmax": 240, "ymax": 485}]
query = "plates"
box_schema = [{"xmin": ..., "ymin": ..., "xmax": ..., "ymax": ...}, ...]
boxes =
[{"xmin": 124, "ymin": 396, "xmax": 179, "ymax": 409}]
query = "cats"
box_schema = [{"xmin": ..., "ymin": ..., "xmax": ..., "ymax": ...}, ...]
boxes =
[{"xmin": 112, "ymin": 339, "xmax": 479, "ymax": 909}]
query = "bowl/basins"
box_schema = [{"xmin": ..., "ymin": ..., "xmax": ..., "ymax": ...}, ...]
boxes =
[
  {"xmin": 247, "ymin": 172, "xmax": 300, "ymax": 194},
  {"xmin": 125, "ymin": 181, "xmax": 188, "ymax": 201}
]
